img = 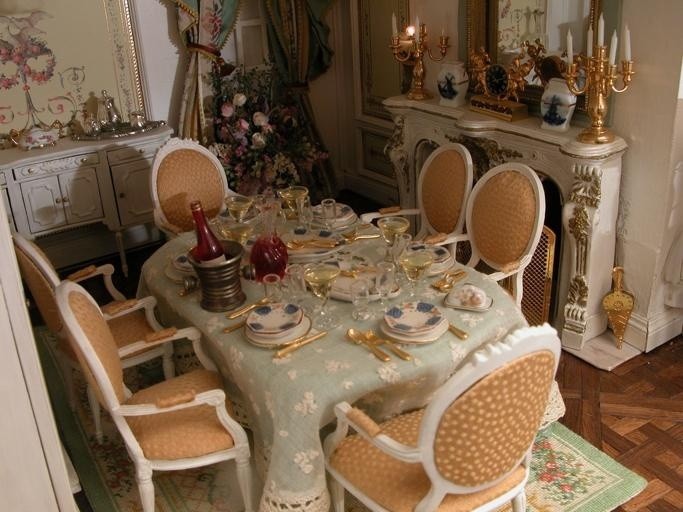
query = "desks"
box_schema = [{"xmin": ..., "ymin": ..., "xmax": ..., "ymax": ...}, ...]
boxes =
[{"xmin": 139, "ymin": 199, "xmax": 566, "ymax": 512}]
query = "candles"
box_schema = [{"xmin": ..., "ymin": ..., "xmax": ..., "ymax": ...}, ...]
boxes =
[
  {"xmin": 567, "ymin": 12, "xmax": 631, "ymax": 65},
  {"xmin": 392, "ymin": 13, "xmax": 446, "ymax": 41}
]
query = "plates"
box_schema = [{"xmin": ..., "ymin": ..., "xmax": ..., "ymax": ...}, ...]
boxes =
[
  {"xmin": 243, "ymin": 302, "xmax": 312, "ymax": 349},
  {"xmin": 378, "ymin": 300, "xmax": 451, "ymax": 344}
]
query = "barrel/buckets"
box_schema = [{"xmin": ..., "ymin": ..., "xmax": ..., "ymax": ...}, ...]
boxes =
[{"xmin": 185, "ymin": 240, "xmax": 249, "ymax": 313}]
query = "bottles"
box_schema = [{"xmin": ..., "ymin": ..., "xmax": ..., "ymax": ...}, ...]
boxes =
[
  {"xmin": 250, "ymin": 208, "xmax": 289, "ymax": 287},
  {"xmin": 188, "ymin": 200, "xmax": 229, "ymax": 263}
]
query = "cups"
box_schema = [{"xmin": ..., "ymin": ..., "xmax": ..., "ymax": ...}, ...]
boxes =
[
  {"xmin": 128, "ymin": 111, "xmax": 148, "ymax": 132},
  {"xmin": 78, "ymin": 112, "xmax": 101, "ymax": 137}
]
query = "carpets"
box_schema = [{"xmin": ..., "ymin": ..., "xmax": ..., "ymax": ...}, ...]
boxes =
[{"xmin": 33, "ymin": 290, "xmax": 649, "ymax": 512}]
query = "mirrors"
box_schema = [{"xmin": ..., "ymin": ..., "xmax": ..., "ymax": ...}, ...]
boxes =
[{"xmin": 458, "ymin": 1, "xmax": 602, "ymax": 116}]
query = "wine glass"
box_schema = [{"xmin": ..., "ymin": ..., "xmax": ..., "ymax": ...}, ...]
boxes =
[
  {"xmin": 399, "ymin": 249, "xmax": 432, "ymax": 301},
  {"xmin": 348, "ymin": 279, "xmax": 373, "ymax": 321},
  {"xmin": 303, "ymin": 265, "xmax": 343, "ymax": 332}
]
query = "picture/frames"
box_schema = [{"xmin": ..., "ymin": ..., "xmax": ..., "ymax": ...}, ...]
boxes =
[
  {"xmin": 0, "ymin": 1, "xmax": 152, "ymax": 149},
  {"xmin": 498, "ymin": 0, "xmax": 549, "ymax": 86}
]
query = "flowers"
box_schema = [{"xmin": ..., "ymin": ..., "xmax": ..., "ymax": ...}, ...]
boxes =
[{"xmin": 0, "ymin": 12, "xmax": 85, "ymax": 134}]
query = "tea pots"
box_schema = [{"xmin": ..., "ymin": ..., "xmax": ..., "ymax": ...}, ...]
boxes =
[{"xmin": 96, "ymin": 88, "xmax": 122, "ymax": 132}]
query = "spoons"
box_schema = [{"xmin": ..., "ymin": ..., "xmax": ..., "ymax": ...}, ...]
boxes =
[
  {"xmin": 431, "ymin": 268, "xmax": 468, "ymax": 293},
  {"xmin": 346, "ymin": 326, "xmax": 413, "ymax": 362}
]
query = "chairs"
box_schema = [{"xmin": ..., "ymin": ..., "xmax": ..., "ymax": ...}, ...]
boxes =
[
  {"xmin": 408, "ymin": 161, "xmax": 546, "ymax": 324},
  {"xmin": 321, "ymin": 323, "xmax": 559, "ymax": 512},
  {"xmin": 359, "ymin": 141, "xmax": 474, "ymax": 271},
  {"xmin": 147, "ymin": 138, "xmax": 246, "ymax": 244},
  {"xmin": 51, "ymin": 278, "xmax": 256, "ymax": 512},
  {"xmin": 9, "ymin": 232, "xmax": 177, "ymax": 450}
]
